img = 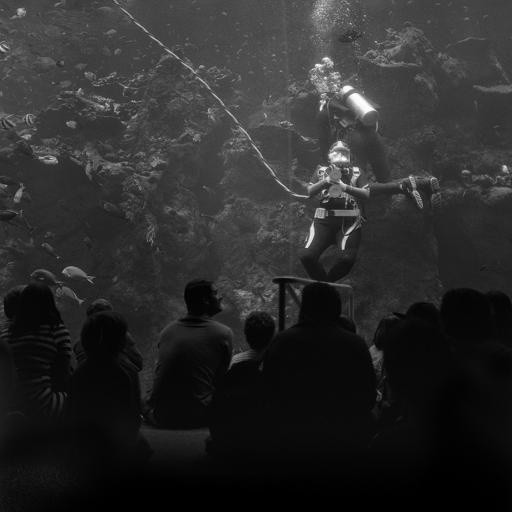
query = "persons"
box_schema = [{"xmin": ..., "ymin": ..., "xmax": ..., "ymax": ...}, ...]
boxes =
[
  {"xmin": 0, "ymin": 278, "xmax": 512, "ymax": 512},
  {"xmin": 299, "ymin": 129, "xmax": 371, "ymax": 282},
  {"xmin": 316, "ymin": 83, "xmax": 439, "ymax": 211}
]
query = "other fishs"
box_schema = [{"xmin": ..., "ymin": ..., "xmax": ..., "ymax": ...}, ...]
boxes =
[
  {"xmin": 339, "ymin": 30, "xmax": 364, "ymax": 44},
  {"xmin": 0, "ymin": 0, "xmax": 125, "ymax": 307}
]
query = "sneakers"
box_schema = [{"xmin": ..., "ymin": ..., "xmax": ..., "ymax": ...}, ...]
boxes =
[{"xmin": 401, "ymin": 176, "xmax": 441, "ymax": 212}]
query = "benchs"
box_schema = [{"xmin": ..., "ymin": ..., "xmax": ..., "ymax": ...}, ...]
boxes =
[{"xmin": 273, "ymin": 276, "xmax": 355, "ymax": 336}]
